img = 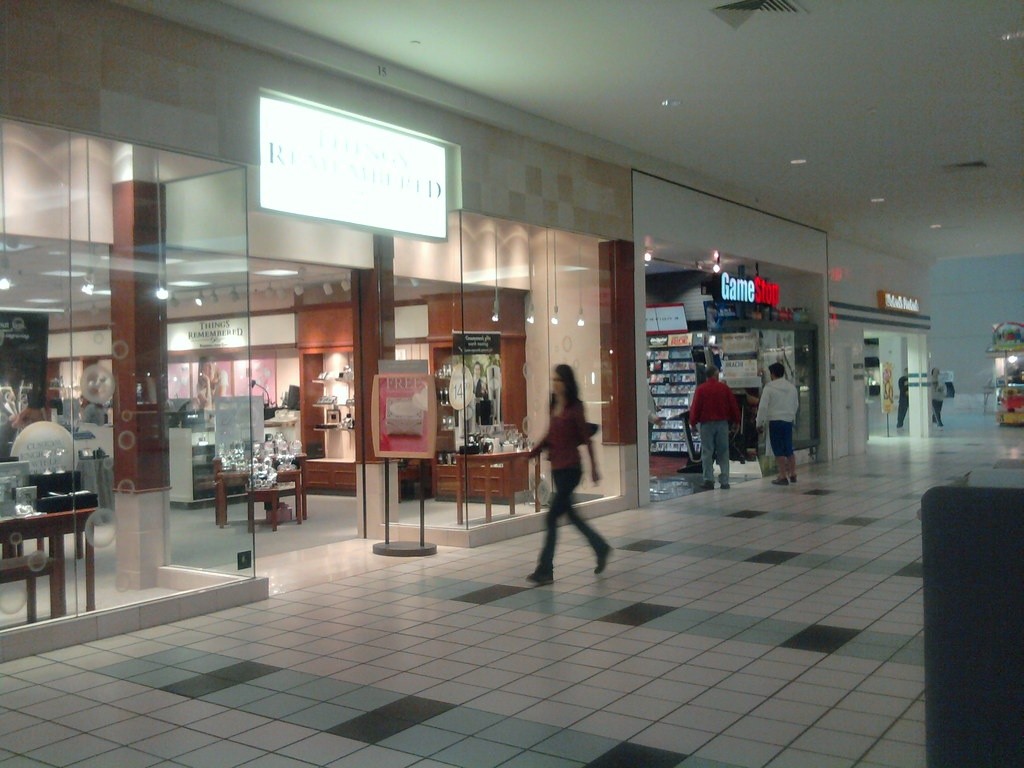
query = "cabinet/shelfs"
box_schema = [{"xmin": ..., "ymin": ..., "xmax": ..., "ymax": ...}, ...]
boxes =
[
  {"xmin": 644, "ymin": 333, "xmax": 712, "ymax": 455},
  {"xmin": 419, "ymin": 289, "xmax": 530, "ymax": 504},
  {"xmin": 294, "ymin": 301, "xmax": 360, "ymax": 496}
]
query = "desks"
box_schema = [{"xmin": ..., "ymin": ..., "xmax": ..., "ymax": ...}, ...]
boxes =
[
  {"xmin": 453, "ymin": 450, "xmax": 542, "ymax": 524},
  {"xmin": 213, "ymin": 452, "xmax": 307, "ymax": 525},
  {"xmin": 0, "ymin": 507, "xmax": 96, "ymax": 613},
  {"xmin": 216, "ymin": 467, "xmax": 303, "ymax": 524}
]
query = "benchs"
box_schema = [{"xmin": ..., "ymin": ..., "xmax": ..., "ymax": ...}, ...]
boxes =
[
  {"xmin": 244, "ymin": 484, "xmax": 307, "ymax": 534},
  {"xmin": 0, "ymin": 554, "xmax": 66, "ymax": 620}
]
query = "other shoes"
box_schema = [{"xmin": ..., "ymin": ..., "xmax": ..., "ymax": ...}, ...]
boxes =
[
  {"xmin": 701, "ymin": 481, "xmax": 730, "ymax": 490},
  {"xmin": 595, "ymin": 546, "xmax": 612, "ymax": 575},
  {"xmin": 526, "ymin": 571, "xmax": 553, "ymax": 585},
  {"xmin": 771, "ymin": 474, "xmax": 798, "ymax": 485}
]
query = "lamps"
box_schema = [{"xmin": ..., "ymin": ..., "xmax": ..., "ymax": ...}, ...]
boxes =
[
  {"xmin": 169, "ymin": 277, "xmax": 352, "ymax": 300},
  {"xmin": 155, "ymin": 152, "xmax": 169, "ymax": 300},
  {"xmin": 1, "ymin": 121, "xmax": 11, "ymax": 290},
  {"xmin": 491, "ymin": 221, "xmax": 499, "ymax": 321},
  {"xmin": 551, "ymin": 232, "xmax": 560, "ymax": 325},
  {"xmin": 82, "ymin": 137, "xmax": 95, "ymax": 296},
  {"xmin": 526, "ymin": 228, "xmax": 536, "ymax": 324},
  {"xmin": 576, "ymin": 237, "xmax": 584, "ymax": 327}
]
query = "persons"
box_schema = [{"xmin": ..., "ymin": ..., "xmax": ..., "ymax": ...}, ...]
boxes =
[
  {"xmin": 689, "ymin": 364, "xmax": 741, "ymax": 489},
  {"xmin": 896, "ymin": 368, "xmax": 908, "ymax": 428},
  {"xmin": 471, "ymin": 361, "xmax": 482, "ymax": 426},
  {"xmin": 647, "ymin": 360, "xmax": 665, "ymax": 451},
  {"xmin": 755, "ymin": 363, "xmax": 799, "ymax": 485},
  {"xmin": 80, "ymin": 394, "xmax": 105, "ymax": 426},
  {"xmin": 520, "ymin": 364, "xmax": 614, "ymax": 585},
  {"xmin": 196, "ymin": 356, "xmax": 228, "ymax": 409},
  {"xmin": 930, "ymin": 367, "xmax": 946, "ymax": 430}
]
